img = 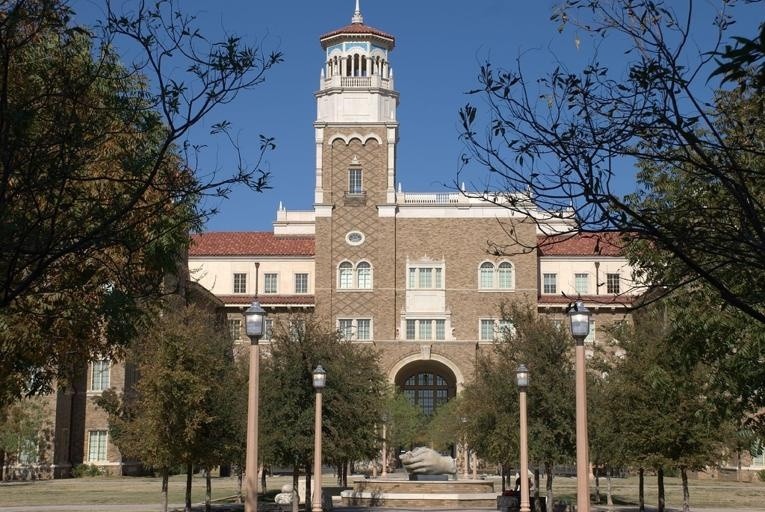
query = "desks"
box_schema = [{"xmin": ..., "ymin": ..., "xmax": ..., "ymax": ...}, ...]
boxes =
[{"xmin": 497, "ymin": 496, "xmax": 546, "ymax": 512}]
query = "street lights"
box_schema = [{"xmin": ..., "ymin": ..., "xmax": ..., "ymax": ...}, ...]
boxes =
[
  {"xmin": 461, "ymin": 415, "xmax": 468, "ymax": 478},
  {"xmin": 240, "ymin": 300, "xmax": 265, "ymax": 512},
  {"xmin": 309, "ymin": 363, "xmax": 328, "ymax": 511},
  {"xmin": 515, "ymin": 362, "xmax": 535, "ymax": 506},
  {"xmin": 562, "ymin": 297, "xmax": 592, "ymax": 510},
  {"xmin": 381, "ymin": 412, "xmax": 389, "ymax": 478}
]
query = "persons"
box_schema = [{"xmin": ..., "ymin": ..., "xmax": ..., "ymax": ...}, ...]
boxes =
[
  {"xmin": 399, "ymin": 446, "xmax": 457, "ymax": 475},
  {"xmin": 515, "ymin": 471, "xmax": 533, "ymax": 511}
]
